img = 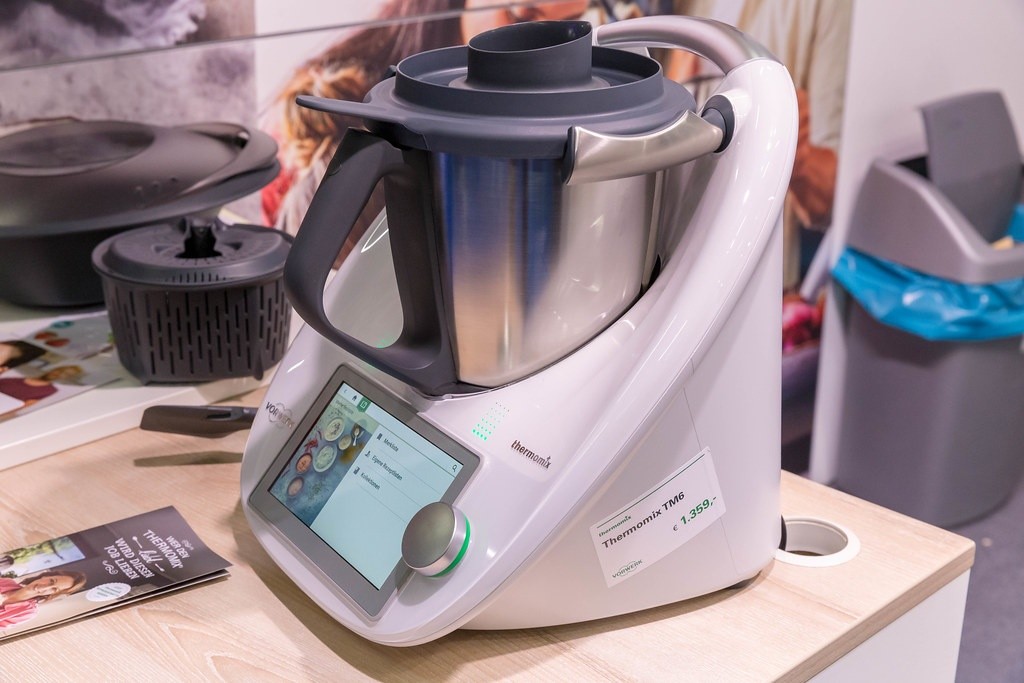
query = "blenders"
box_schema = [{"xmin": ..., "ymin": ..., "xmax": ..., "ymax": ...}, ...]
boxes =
[{"xmin": 241, "ymin": 16, "xmax": 849, "ymax": 650}]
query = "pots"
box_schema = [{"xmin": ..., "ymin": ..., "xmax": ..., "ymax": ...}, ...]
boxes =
[
  {"xmin": 90, "ymin": 216, "xmax": 296, "ymax": 385},
  {"xmin": 0, "ymin": 117, "xmax": 281, "ymax": 310}
]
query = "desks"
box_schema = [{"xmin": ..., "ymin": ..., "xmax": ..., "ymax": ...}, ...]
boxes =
[{"xmin": 2, "ymin": 221, "xmax": 982, "ymax": 683}]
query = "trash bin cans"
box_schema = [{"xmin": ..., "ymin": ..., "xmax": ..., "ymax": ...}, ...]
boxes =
[{"xmin": 827, "ymin": 87, "xmax": 1024, "ymax": 530}]
